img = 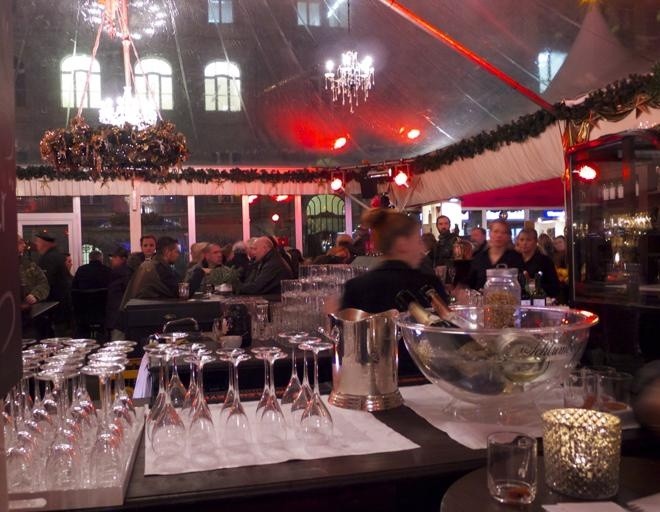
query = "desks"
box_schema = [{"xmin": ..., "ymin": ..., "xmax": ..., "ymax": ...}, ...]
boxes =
[
  {"xmin": 22, "ymin": 298, "xmax": 61, "ymax": 335},
  {"xmin": 123, "ymin": 293, "xmax": 280, "ymax": 357}
]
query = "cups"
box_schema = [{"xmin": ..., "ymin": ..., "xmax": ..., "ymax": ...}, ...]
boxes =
[
  {"xmin": 264, "ymin": 264, "xmax": 368, "ymax": 340},
  {"xmin": 486, "ymin": 432, "xmax": 537, "ymax": 507},
  {"xmin": 563, "ymin": 366, "xmax": 633, "ymax": 414},
  {"xmin": 178, "ymin": 283, "xmax": 190, "ymax": 300}
]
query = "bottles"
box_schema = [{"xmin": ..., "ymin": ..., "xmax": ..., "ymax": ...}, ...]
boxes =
[
  {"xmin": 599, "ymin": 177, "xmax": 640, "ymax": 200},
  {"xmin": 396, "ymin": 267, "xmax": 522, "ymax": 395}
]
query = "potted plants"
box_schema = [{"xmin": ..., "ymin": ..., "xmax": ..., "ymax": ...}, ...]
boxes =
[{"xmin": 200, "ymin": 264, "xmax": 243, "ymax": 296}]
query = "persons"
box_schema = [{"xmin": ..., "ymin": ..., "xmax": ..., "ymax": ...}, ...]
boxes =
[
  {"xmin": 328, "ymin": 209, "xmax": 568, "ymax": 377},
  {"xmin": 16, "ymin": 227, "xmax": 299, "ymax": 349}
]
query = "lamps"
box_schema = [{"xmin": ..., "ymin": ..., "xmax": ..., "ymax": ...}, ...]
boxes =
[
  {"xmin": 330, "ymin": 169, "xmax": 347, "ymax": 196},
  {"xmin": 601, "ymin": 212, "xmax": 654, "ymax": 284},
  {"xmin": 393, "ymin": 164, "xmax": 414, "ymax": 192},
  {"xmin": 99, "ymin": 0, "xmax": 159, "ymax": 130},
  {"xmin": 323, "ymin": 0, "xmax": 375, "ymax": 115}
]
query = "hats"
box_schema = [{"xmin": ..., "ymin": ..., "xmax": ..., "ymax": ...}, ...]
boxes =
[
  {"xmin": 108, "ymin": 247, "xmax": 128, "ymax": 258},
  {"xmin": 35, "ymin": 232, "xmax": 56, "ymax": 242},
  {"xmin": 156, "ymin": 237, "xmax": 178, "ymax": 249}
]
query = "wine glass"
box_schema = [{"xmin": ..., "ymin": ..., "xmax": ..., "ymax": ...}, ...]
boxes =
[
  {"xmin": 146, "ymin": 333, "xmax": 334, "ymax": 492},
  {"xmin": 4, "ymin": 330, "xmax": 147, "ymax": 491}
]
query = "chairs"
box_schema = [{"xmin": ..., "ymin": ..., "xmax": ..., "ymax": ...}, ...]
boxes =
[{"xmin": 69, "ymin": 287, "xmax": 106, "ymax": 339}]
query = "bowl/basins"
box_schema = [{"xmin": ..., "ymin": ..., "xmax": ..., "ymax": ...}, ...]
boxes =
[{"xmin": 392, "ymin": 306, "xmax": 600, "ymax": 405}]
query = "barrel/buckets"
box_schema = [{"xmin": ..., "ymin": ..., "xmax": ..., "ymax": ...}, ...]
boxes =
[{"xmin": 316, "ymin": 308, "xmax": 407, "ymax": 412}]
